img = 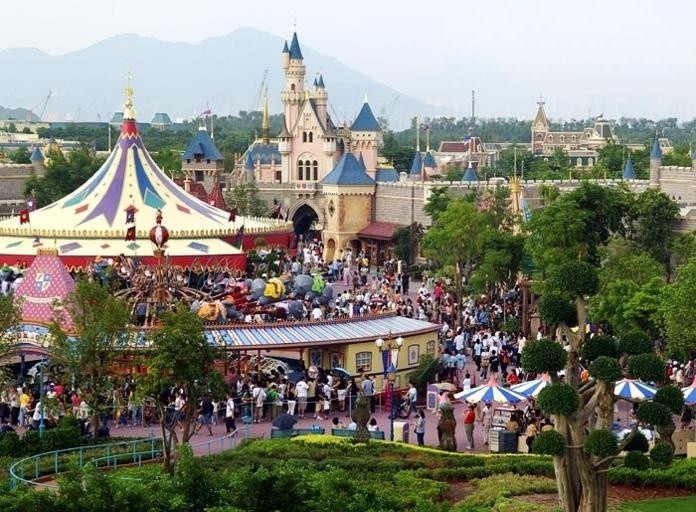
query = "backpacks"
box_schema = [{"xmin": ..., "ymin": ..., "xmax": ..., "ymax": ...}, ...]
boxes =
[{"xmin": 267, "ymin": 391, "xmax": 273, "ymax": 401}]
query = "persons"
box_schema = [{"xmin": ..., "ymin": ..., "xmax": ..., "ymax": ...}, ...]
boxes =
[
  {"xmin": 581, "ymin": 366, "xmax": 590, "ymax": 381},
  {"xmin": 582, "ymin": 412, "xmax": 655, "ymax": 441},
  {"xmin": 414, "ymin": 260, "xmax": 563, "ymax": 454},
  {"xmin": 1, "ymin": 365, "xmax": 426, "ymax": 451},
  {"xmin": 70, "ymin": 230, "xmax": 415, "ymax": 323},
  {"xmin": 681, "ymin": 404, "xmax": 692, "ymax": 430},
  {"xmin": 667, "ymin": 358, "xmax": 695, "ymax": 388},
  {"xmin": 1, "ymin": 260, "xmax": 28, "ymax": 278}
]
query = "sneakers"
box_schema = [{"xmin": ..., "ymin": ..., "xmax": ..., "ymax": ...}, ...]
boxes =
[
  {"xmin": 480, "ymin": 376, "xmax": 487, "ymax": 382},
  {"xmin": 106, "ymin": 415, "xmax": 330, "ymax": 437},
  {"xmin": 403, "ymin": 415, "xmax": 421, "ymax": 420},
  {"xmin": 466, "ymin": 446, "xmax": 474, "ymax": 451}
]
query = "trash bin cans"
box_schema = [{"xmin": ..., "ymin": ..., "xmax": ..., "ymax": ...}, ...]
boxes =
[
  {"xmin": 393, "ymin": 421, "xmax": 409, "ymax": 443},
  {"xmin": 426, "ymin": 382, "xmax": 457, "ymax": 411}
]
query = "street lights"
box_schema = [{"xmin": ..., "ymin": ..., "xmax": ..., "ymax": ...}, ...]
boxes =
[{"xmin": 375, "ymin": 329, "xmax": 404, "ymax": 441}]
